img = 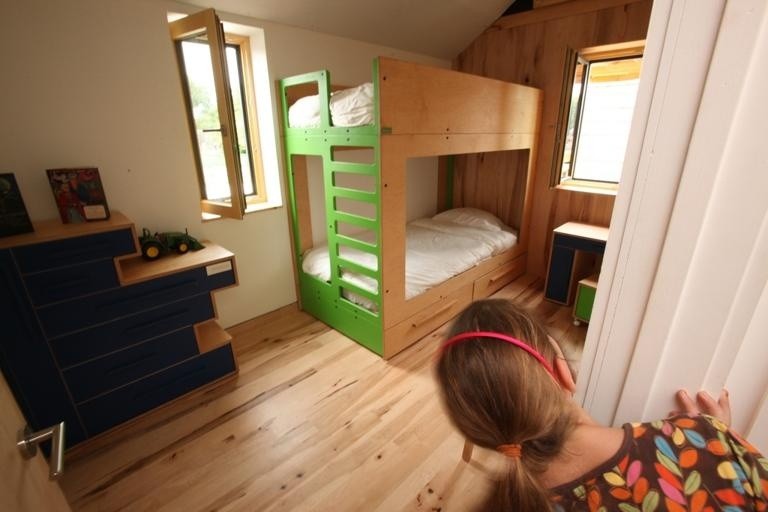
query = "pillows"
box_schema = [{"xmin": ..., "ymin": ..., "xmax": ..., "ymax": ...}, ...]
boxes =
[{"xmin": 432, "ymin": 207, "xmax": 505, "ymax": 232}]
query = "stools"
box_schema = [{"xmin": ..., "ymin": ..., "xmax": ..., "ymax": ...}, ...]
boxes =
[{"xmin": 572, "ymin": 275, "xmax": 599, "ymax": 326}]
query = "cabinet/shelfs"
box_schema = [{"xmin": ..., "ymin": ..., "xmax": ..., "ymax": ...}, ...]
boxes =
[{"xmin": 1, "ymin": 211, "xmax": 240, "ymax": 459}]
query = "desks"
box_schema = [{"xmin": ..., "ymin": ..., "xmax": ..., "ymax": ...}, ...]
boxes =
[{"xmin": 542, "ymin": 222, "xmax": 609, "ymax": 307}]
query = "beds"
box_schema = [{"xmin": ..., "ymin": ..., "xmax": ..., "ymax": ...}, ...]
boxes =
[{"xmin": 274, "ymin": 55, "xmax": 544, "ymax": 360}]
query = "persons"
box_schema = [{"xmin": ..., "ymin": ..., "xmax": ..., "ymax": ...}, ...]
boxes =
[{"xmin": 434, "ymin": 299, "xmax": 768, "ymax": 512}]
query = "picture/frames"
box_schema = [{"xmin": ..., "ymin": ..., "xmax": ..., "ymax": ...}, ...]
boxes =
[
  {"xmin": 46, "ymin": 167, "xmax": 111, "ymax": 223},
  {"xmin": 0, "ymin": 172, "xmax": 35, "ymax": 239}
]
